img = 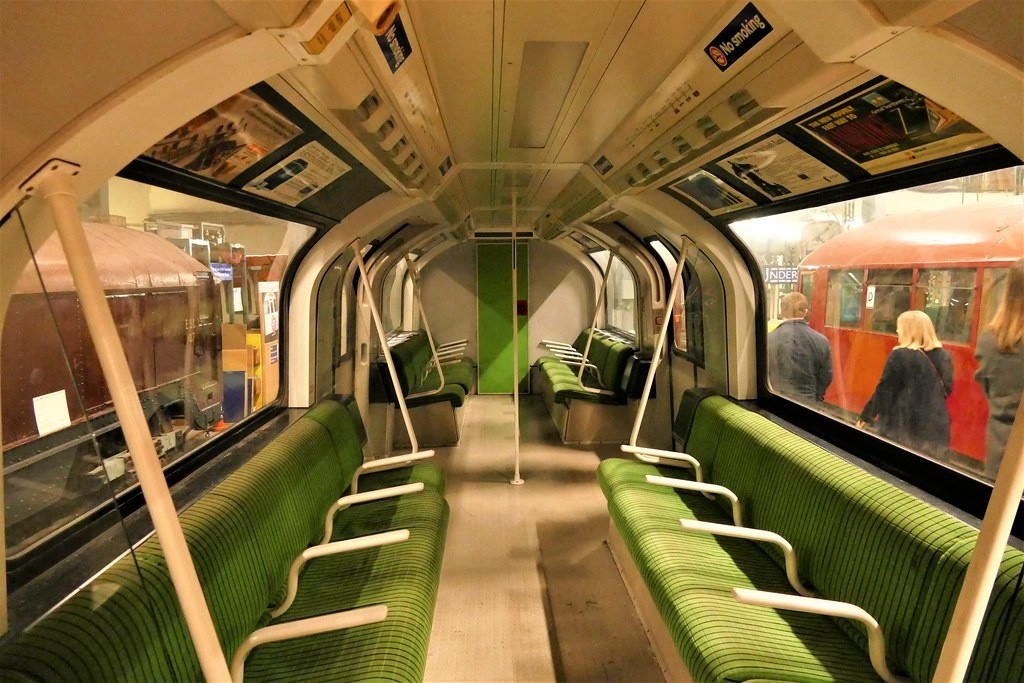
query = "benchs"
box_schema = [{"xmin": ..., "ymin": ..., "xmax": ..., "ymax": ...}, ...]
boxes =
[
  {"xmin": 539, "ymin": 325, "xmax": 646, "ymax": 444},
  {"xmin": 597, "ymin": 388, "xmax": 1024, "ymax": 683},
  {"xmin": 376, "ymin": 327, "xmax": 477, "ymax": 447},
  {"xmin": 0, "ymin": 392, "xmax": 448, "ymax": 683}
]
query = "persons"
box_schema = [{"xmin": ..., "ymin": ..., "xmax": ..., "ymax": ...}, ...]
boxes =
[
  {"xmin": 855, "ymin": 310, "xmax": 955, "ymax": 465},
  {"xmin": 765, "ymin": 291, "xmax": 833, "ymax": 403},
  {"xmin": 972, "ymin": 257, "xmax": 1024, "ymax": 481}
]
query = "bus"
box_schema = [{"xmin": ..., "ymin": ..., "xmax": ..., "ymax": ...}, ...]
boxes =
[
  {"xmin": 1, "ymin": 220, "xmax": 225, "ymax": 529},
  {"xmin": 615, "ymin": 214, "xmax": 846, "ymax": 357},
  {"xmin": 795, "ymin": 197, "xmax": 1024, "ymax": 498}
]
